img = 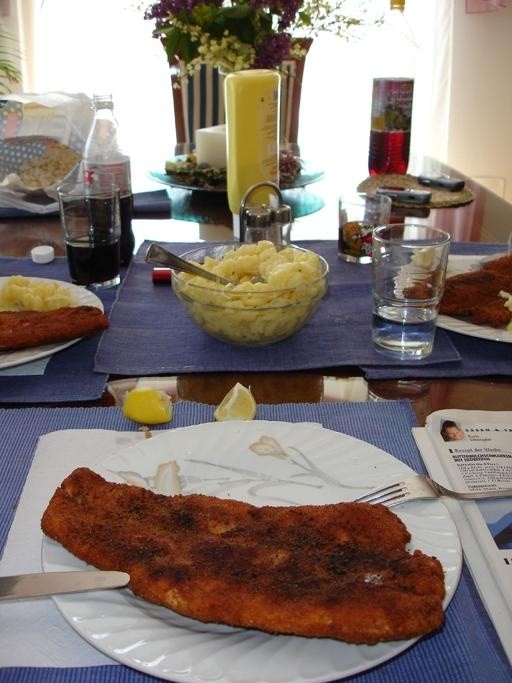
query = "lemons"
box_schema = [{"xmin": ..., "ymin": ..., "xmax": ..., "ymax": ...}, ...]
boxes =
[
  {"xmin": 214, "ymin": 383, "xmax": 255, "ymax": 421},
  {"xmin": 124, "ymin": 389, "xmax": 172, "ymax": 424}
]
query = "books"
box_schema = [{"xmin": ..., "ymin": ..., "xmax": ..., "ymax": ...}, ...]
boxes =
[{"xmin": 410, "ymin": 406, "xmax": 512, "ymax": 664}]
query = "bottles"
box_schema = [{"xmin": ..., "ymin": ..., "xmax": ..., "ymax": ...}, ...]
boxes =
[
  {"xmin": 243, "ymin": 203, "xmax": 292, "ymax": 246},
  {"xmin": 368, "ymin": 1, "xmax": 415, "ymax": 187},
  {"xmin": 82, "ymin": 94, "xmax": 135, "ymax": 271}
]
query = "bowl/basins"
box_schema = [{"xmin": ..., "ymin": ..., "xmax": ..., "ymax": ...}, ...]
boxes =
[{"xmin": 170, "ymin": 238, "xmax": 331, "ymax": 349}]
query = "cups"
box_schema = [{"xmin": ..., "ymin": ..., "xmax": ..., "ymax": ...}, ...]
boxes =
[
  {"xmin": 338, "ymin": 192, "xmax": 393, "ymax": 266},
  {"xmin": 371, "ymin": 222, "xmax": 452, "ymax": 363},
  {"xmin": 57, "ymin": 180, "xmax": 123, "ymax": 291}
]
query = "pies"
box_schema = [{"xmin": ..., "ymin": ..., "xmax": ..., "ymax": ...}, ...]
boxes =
[{"xmin": 21, "ymin": 144, "xmax": 79, "ymax": 188}]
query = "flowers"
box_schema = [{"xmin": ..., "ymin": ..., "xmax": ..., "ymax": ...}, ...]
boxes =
[{"xmin": 137, "ymin": 1, "xmax": 386, "ymax": 90}]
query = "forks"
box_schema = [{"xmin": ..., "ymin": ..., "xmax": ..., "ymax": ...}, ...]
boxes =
[{"xmin": 352, "ymin": 475, "xmax": 512, "ymax": 509}]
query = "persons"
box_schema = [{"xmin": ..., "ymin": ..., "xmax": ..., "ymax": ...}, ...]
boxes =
[{"xmin": 440, "ymin": 419, "xmax": 465, "ymax": 442}]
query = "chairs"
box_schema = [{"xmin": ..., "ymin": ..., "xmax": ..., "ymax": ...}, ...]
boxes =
[{"xmin": 171, "ymin": 38, "xmax": 314, "ymax": 156}]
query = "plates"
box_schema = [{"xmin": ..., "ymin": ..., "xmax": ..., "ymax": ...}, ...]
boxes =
[
  {"xmin": 0, "ymin": 274, "xmax": 106, "ymax": 370},
  {"xmin": 42, "ymin": 418, "xmax": 464, "ymax": 682},
  {"xmin": 397, "ymin": 253, "xmax": 512, "ymax": 342}
]
